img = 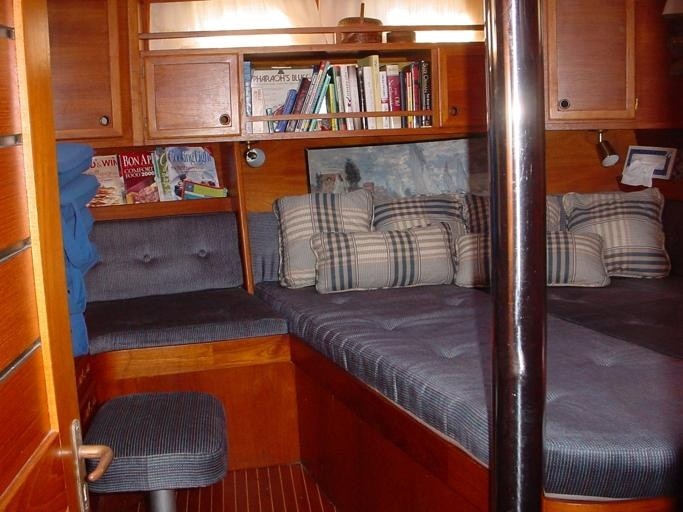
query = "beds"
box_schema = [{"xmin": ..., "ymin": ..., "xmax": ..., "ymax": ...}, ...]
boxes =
[{"xmin": 240, "ymin": 130, "xmax": 683, "ymax": 512}]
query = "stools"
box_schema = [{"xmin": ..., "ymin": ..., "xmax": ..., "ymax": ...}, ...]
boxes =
[{"xmin": 85, "ymin": 391, "xmax": 229, "ymax": 511}]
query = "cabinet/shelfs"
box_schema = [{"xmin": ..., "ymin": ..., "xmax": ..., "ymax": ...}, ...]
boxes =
[
  {"xmin": 138, "ymin": 0, "xmax": 485, "ymax": 141},
  {"xmin": 47, "ymin": 0, "xmax": 122, "ymax": 140},
  {"xmin": 546, "ymin": 0, "xmax": 637, "ymax": 122}
]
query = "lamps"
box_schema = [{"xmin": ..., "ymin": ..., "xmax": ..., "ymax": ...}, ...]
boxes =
[
  {"xmin": 593, "ymin": 129, "xmax": 619, "ymax": 167},
  {"xmin": 241, "ymin": 141, "xmax": 266, "ymax": 169}
]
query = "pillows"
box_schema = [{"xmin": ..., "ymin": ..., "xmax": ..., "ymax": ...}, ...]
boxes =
[
  {"xmin": 309, "ymin": 222, "xmax": 457, "ymax": 295},
  {"xmin": 273, "ymin": 189, "xmax": 374, "ymax": 290},
  {"xmin": 455, "ymin": 231, "xmax": 610, "ymax": 288},
  {"xmin": 465, "ymin": 190, "xmax": 567, "ymax": 232},
  {"xmin": 563, "ymin": 186, "xmax": 671, "ymax": 279},
  {"xmin": 374, "ymin": 192, "xmax": 470, "ymax": 249}
]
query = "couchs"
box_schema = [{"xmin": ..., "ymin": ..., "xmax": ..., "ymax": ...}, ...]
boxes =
[{"xmin": 85, "ymin": 212, "xmax": 300, "ymax": 470}]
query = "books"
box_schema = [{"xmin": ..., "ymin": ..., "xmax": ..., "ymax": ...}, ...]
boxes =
[
  {"xmin": 243, "ymin": 54, "xmax": 432, "ymax": 133},
  {"xmin": 84, "ymin": 145, "xmax": 228, "ymax": 208}
]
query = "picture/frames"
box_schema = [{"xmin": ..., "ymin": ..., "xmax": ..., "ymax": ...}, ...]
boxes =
[{"xmin": 305, "ymin": 134, "xmax": 489, "ymax": 205}]
